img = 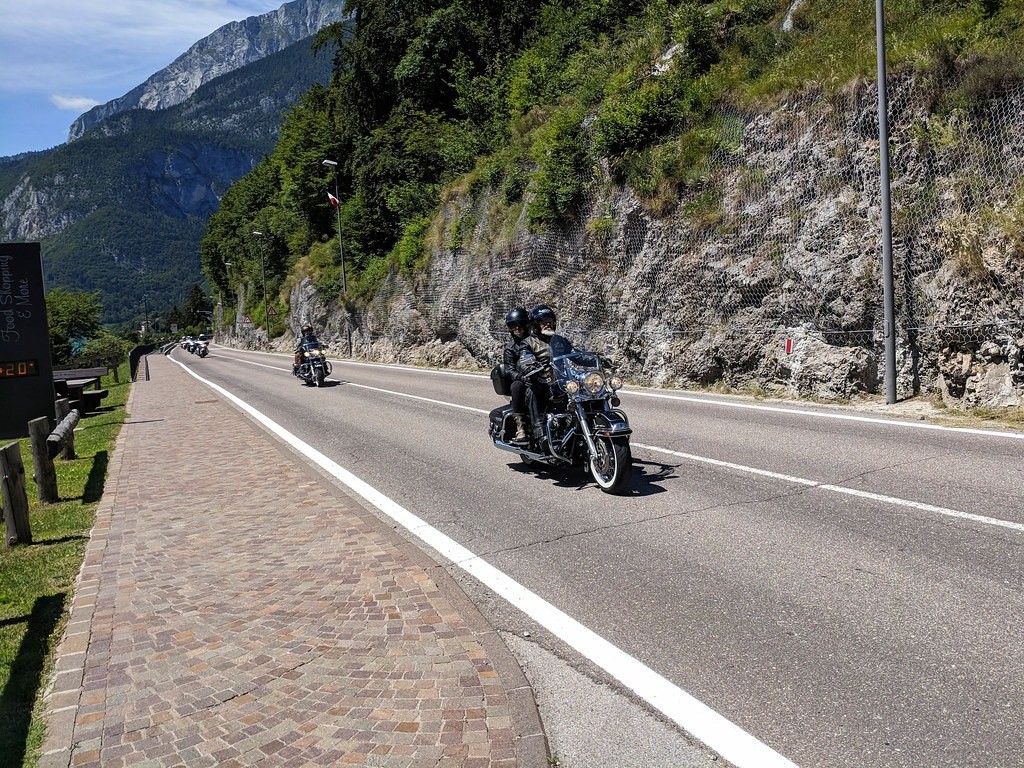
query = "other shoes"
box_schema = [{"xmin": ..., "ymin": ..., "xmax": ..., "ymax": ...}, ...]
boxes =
[{"xmin": 298, "ymin": 368, "xmax": 306, "ymax": 374}]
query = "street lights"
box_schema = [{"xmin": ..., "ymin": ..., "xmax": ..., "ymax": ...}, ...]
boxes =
[
  {"xmin": 223, "ymin": 262, "xmax": 236, "ymax": 328},
  {"xmin": 322, "ymin": 159, "xmax": 353, "ymax": 361},
  {"xmin": 253, "ymin": 231, "xmax": 269, "ymax": 341}
]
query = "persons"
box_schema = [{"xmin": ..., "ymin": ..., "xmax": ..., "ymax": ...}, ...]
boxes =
[
  {"xmin": 520, "ymin": 307, "xmax": 613, "ymax": 455},
  {"xmin": 295, "ymin": 325, "xmax": 329, "ymax": 368},
  {"xmin": 198, "ymin": 334, "xmax": 206, "ymax": 341},
  {"xmin": 503, "ymin": 308, "xmax": 537, "ymax": 443}
]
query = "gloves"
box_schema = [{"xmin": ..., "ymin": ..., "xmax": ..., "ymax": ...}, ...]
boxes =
[
  {"xmin": 519, "ymin": 376, "xmax": 532, "ymax": 387},
  {"xmin": 600, "ymin": 357, "xmax": 612, "ymax": 369}
]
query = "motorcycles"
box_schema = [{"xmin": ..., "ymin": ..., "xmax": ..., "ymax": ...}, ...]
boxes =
[
  {"xmin": 482, "ymin": 328, "xmax": 632, "ymax": 494},
  {"xmin": 180, "ymin": 337, "xmax": 209, "ymax": 358},
  {"xmin": 291, "ymin": 337, "xmax": 331, "ymax": 387}
]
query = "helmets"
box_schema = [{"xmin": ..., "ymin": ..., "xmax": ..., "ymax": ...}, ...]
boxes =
[
  {"xmin": 506, "ymin": 308, "xmax": 531, "ymax": 340},
  {"xmin": 528, "ymin": 305, "xmax": 557, "ymax": 342},
  {"xmin": 182, "ymin": 334, "xmax": 206, "ymax": 341},
  {"xmin": 301, "ymin": 324, "xmax": 314, "ymax": 336}
]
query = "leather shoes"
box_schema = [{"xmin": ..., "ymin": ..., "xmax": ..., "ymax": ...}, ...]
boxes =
[{"xmin": 538, "ymin": 435, "xmax": 552, "ymax": 456}]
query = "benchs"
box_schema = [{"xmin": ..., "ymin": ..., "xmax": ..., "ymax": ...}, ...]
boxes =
[
  {"xmin": 53, "ymin": 367, "xmax": 109, "ymax": 407},
  {"xmin": 53, "ymin": 379, "xmax": 80, "ymax": 412}
]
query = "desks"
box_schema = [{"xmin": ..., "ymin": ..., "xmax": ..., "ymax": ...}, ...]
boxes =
[{"xmin": 66, "ymin": 378, "xmax": 98, "ymax": 415}]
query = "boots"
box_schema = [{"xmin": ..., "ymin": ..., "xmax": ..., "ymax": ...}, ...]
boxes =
[{"xmin": 514, "ymin": 416, "xmax": 528, "ymax": 442}]
